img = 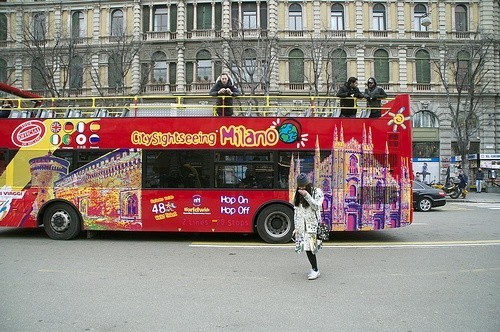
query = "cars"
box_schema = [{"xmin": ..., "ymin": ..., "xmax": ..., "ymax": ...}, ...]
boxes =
[{"xmin": 413, "ymin": 180, "xmax": 447, "ymax": 212}]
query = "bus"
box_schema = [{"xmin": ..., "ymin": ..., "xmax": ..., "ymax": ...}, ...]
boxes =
[{"xmin": 0, "ymin": 94, "xmax": 415, "ymax": 244}]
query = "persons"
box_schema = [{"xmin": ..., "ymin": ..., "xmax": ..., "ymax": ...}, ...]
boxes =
[
  {"xmin": 459, "ymin": 168, "xmax": 484, "ymax": 199},
  {"xmin": 291, "ymin": 173, "xmax": 324, "ymax": 279},
  {"xmin": 336, "ymin": 77, "xmax": 364, "ymax": 117},
  {"xmin": 364, "ymin": 77, "xmax": 387, "ymax": 118},
  {"xmin": 208, "ymin": 73, "xmax": 241, "ymax": 116}
]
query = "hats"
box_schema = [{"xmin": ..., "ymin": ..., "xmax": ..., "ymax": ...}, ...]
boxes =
[{"xmin": 297, "ymin": 173, "xmax": 309, "ymax": 187}]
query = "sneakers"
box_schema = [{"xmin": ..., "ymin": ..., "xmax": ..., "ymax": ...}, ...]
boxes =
[{"xmin": 308, "ymin": 269, "xmax": 320, "ymax": 280}]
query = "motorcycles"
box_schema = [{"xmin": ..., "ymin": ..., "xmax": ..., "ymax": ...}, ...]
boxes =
[{"xmin": 431, "ymin": 179, "xmax": 462, "ymax": 199}]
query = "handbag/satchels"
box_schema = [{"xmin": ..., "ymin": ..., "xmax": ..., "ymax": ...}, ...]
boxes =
[{"xmin": 316, "ymin": 222, "xmax": 330, "ymax": 241}]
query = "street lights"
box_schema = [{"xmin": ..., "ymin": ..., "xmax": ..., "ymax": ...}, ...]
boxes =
[{"xmin": 420, "ymin": 17, "xmax": 432, "ymax": 31}]
